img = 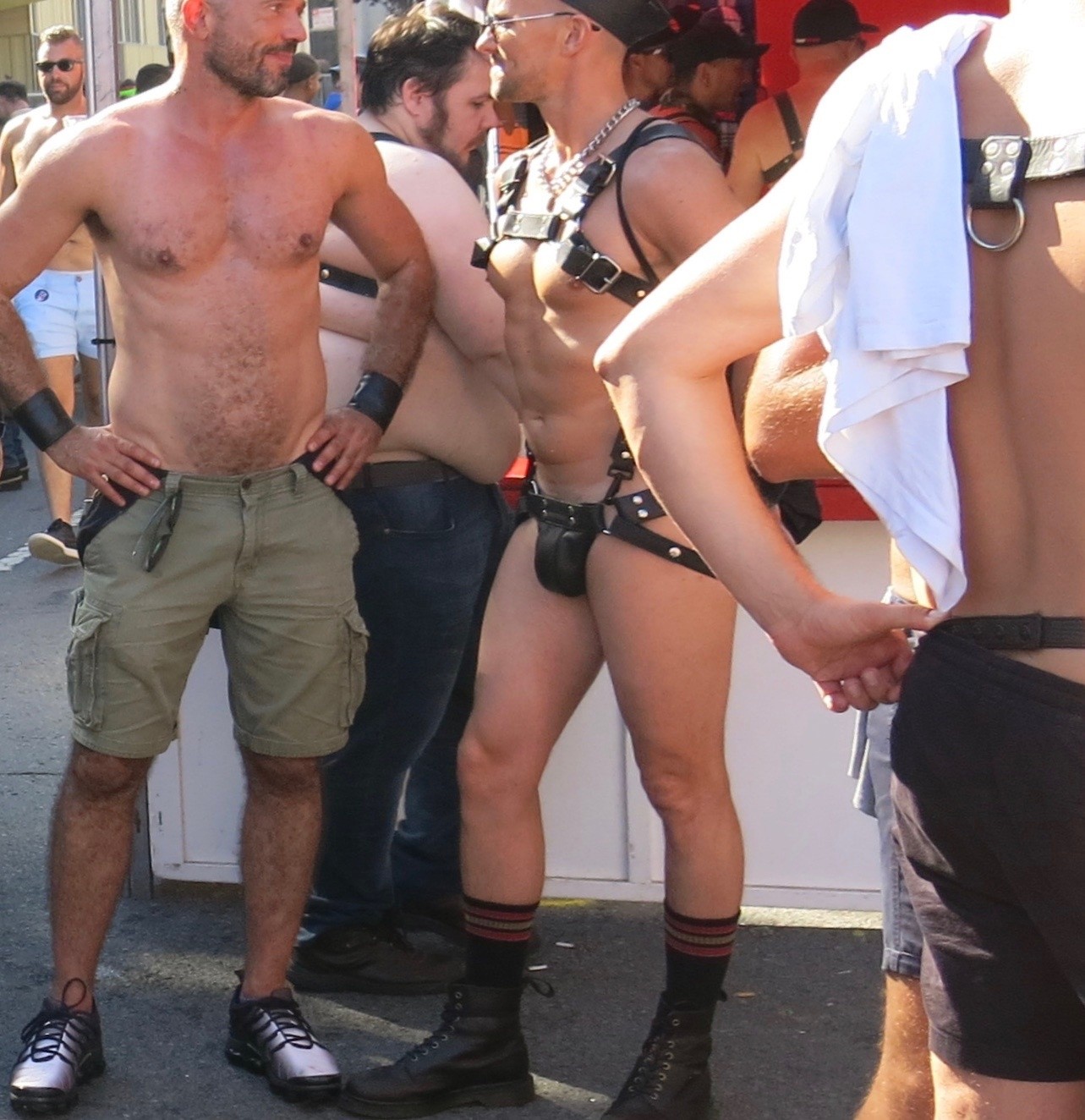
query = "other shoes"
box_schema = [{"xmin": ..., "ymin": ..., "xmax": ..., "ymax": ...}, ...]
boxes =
[{"xmin": 27, "ymin": 520, "xmax": 80, "ymax": 565}]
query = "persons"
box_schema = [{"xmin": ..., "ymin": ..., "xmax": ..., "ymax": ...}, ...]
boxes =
[
  {"xmin": 593, "ymin": 0, "xmax": 1085, "ymax": 1120},
  {"xmin": 1, "ymin": 27, "xmax": 321, "ymax": 563},
  {"xmin": 317, "ymin": 0, "xmax": 766, "ymax": 1120},
  {"xmin": 1, "ymin": 0, "xmax": 885, "ymax": 568},
  {"xmin": 0, "ymin": 0, "xmax": 439, "ymax": 1120}
]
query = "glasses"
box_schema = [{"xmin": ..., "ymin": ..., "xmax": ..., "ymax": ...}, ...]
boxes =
[
  {"xmin": 479, "ymin": 12, "xmax": 601, "ymax": 37},
  {"xmin": 35, "ymin": 60, "xmax": 84, "ymax": 72},
  {"xmin": 132, "ymin": 492, "xmax": 183, "ymax": 573}
]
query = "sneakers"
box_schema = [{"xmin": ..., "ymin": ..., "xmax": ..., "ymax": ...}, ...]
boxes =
[
  {"xmin": 285, "ymin": 916, "xmax": 461, "ymax": 991},
  {"xmin": 224, "ymin": 969, "xmax": 343, "ymax": 1096},
  {"xmin": 430, "ymin": 892, "xmax": 542, "ymax": 956},
  {"xmin": 8, "ymin": 978, "xmax": 105, "ymax": 1110}
]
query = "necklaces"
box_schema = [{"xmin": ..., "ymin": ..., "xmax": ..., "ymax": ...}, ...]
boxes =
[{"xmin": 538, "ymin": 96, "xmax": 641, "ymax": 215}]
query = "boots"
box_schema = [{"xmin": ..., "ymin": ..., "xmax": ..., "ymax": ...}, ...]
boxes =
[
  {"xmin": 600, "ymin": 989, "xmax": 726, "ymax": 1120},
  {"xmin": 340, "ymin": 975, "xmax": 554, "ymax": 1118}
]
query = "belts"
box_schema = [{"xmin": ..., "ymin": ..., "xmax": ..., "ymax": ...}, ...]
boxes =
[{"xmin": 342, "ymin": 458, "xmax": 458, "ymax": 490}]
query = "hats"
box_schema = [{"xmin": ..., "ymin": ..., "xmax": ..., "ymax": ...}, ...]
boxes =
[
  {"xmin": 791, "ymin": 0, "xmax": 881, "ymax": 46},
  {"xmin": 290, "ymin": 52, "xmax": 319, "ymax": 82},
  {"xmin": 562, "ymin": 0, "xmax": 671, "ymax": 49},
  {"xmin": 675, "ymin": 28, "xmax": 770, "ymax": 73}
]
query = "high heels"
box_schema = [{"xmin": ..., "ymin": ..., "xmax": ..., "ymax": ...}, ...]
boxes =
[
  {"xmin": 19, "ymin": 458, "xmax": 29, "ymax": 480},
  {"xmin": 0, "ymin": 467, "xmax": 22, "ymax": 491}
]
